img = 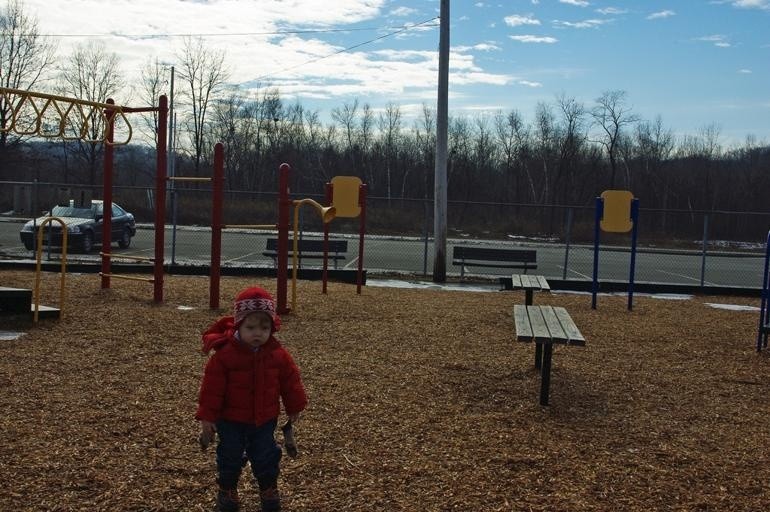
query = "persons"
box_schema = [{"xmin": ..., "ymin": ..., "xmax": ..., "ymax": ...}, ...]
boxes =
[{"xmin": 196, "ymin": 287, "xmax": 307, "ymax": 512}]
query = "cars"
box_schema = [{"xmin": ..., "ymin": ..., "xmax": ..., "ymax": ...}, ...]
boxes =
[{"xmin": 19, "ymin": 196, "xmax": 139, "ymax": 255}]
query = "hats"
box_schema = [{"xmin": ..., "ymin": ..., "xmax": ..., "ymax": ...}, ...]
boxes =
[{"xmin": 233, "ymin": 286, "xmax": 277, "ymax": 331}]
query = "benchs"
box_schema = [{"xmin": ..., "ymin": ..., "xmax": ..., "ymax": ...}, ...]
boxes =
[
  {"xmin": 510, "ymin": 274, "xmax": 551, "ymax": 343},
  {"xmin": 451, "ymin": 244, "xmax": 539, "ymax": 287},
  {"xmin": 264, "ymin": 233, "xmax": 348, "ymax": 266},
  {"xmin": 512, "ymin": 304, "xmax": 587, "ymax": 407}
]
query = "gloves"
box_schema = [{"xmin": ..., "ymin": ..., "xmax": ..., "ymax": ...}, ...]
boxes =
[
  {"xmin": 198, "ymin": 427, "xmax": 215, "ymax": 450},
  {"xmin": 280, "ymin": 418, "xmax": 297, "ymax": 458}
]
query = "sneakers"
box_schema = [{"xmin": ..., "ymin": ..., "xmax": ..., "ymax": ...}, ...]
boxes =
[
  {"xmin": 260, "ymin": 480, "xmax": 280, "ymax": 504},
  {"xmin": 216, "ymin": 488, "xmax": 241, "ymax": 512}
]
